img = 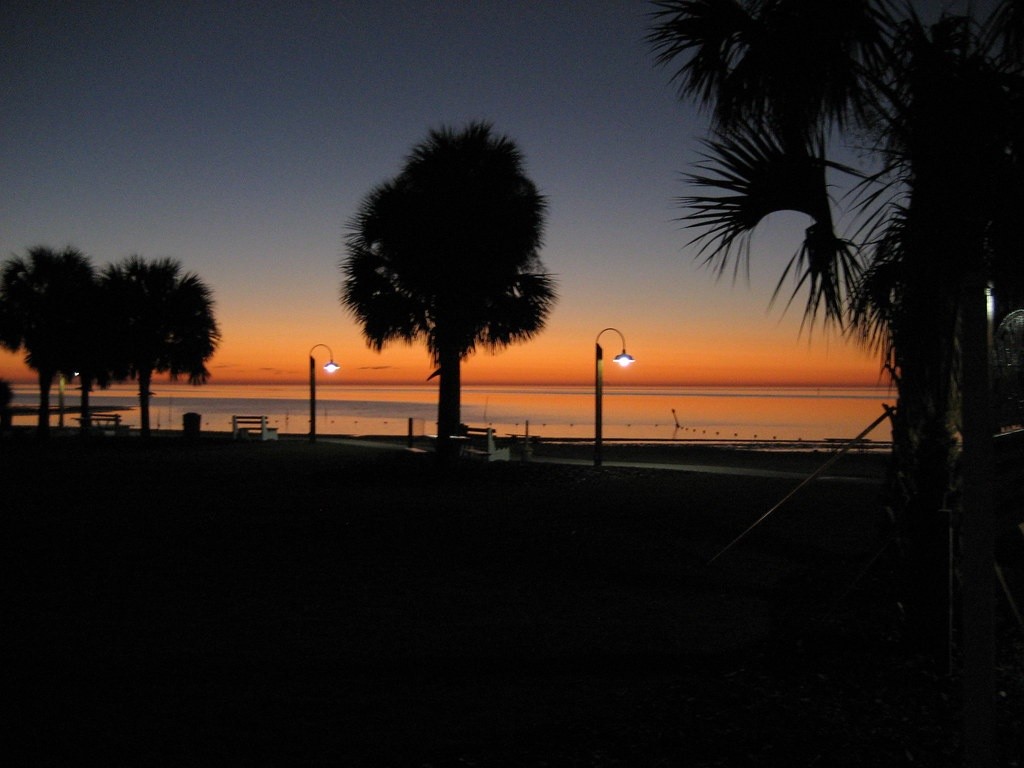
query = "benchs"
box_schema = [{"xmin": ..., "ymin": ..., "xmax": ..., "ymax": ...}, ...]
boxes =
[
  {"xmin": 506, "ymin": 434, "xmax": 552, "ymax": 456},
  {"xmin": 824, "ymin": 438, "xmax": 872, "ymax": 453},
  {"xmin": 461, "ymin": 422, "xmax": 510, "ymax": 462},
  {"xmin": 89, "ymin": 413, "xmax": 130, "ymax": 434},
  {"xmin": 231, "ymin": 415, "xmax": 278, "ymax": 441}
]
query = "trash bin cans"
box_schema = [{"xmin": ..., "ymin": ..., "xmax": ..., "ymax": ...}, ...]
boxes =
[{"xmin": 182, "ymin": 412, "xmax": 201, "ymax": 436}]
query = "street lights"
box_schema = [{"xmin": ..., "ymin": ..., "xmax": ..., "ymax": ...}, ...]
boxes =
[
  {"xmin": 58, "ymin": 373, "xmax": 79, "ymax": 425},
  {"xmin": 309, "ymin": 344, "xmax": 341, "ymax": 437},
  {"xmin": 593, "ymin": 327, "xmax": 636, "ymax": 465}
]
